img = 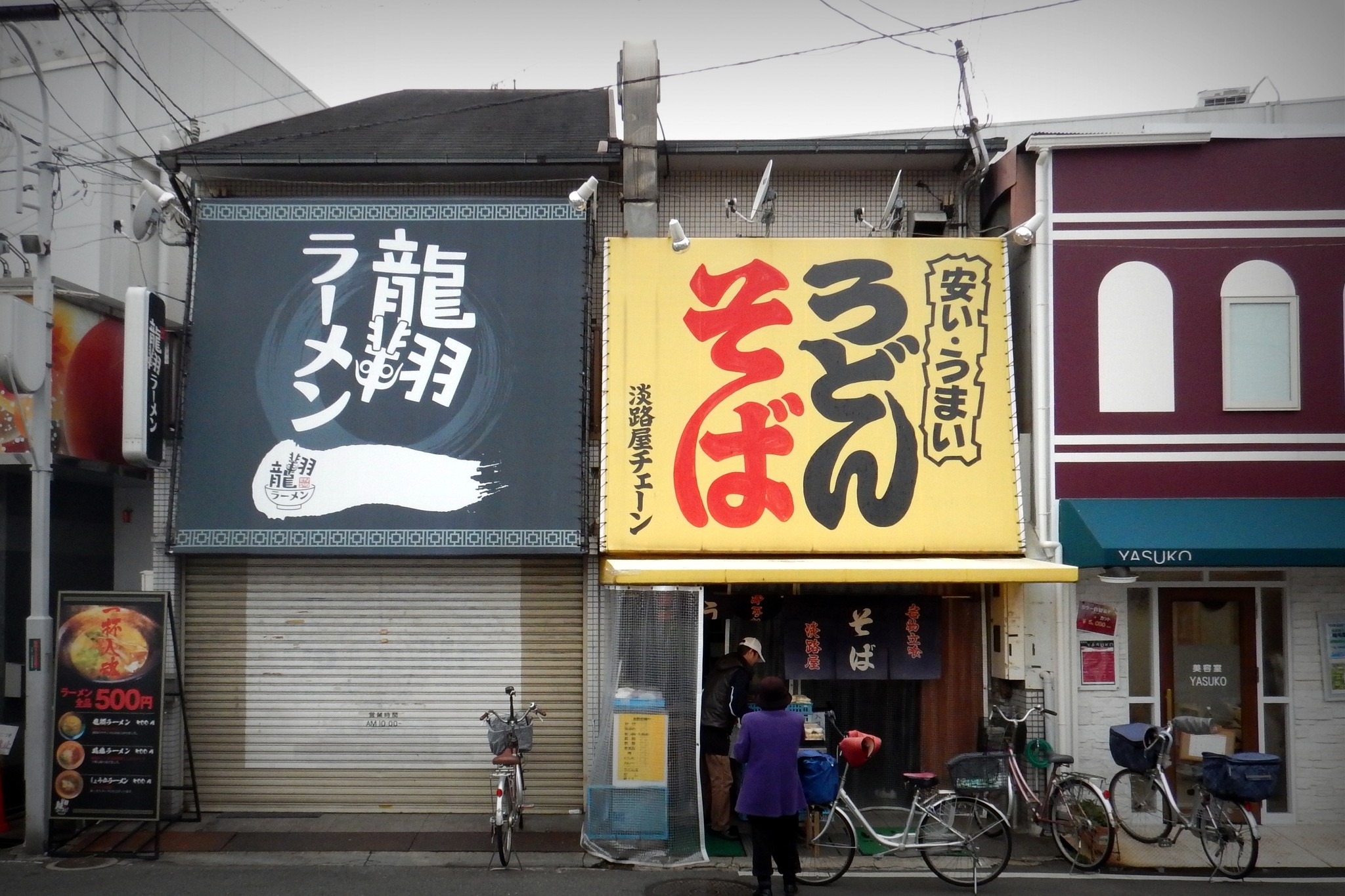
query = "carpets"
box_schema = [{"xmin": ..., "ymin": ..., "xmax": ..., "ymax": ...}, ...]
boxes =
[
  {"xmin": 667, "ymin": 822, "xmax": 747, "ymax": 857},
  {"xmin": 844, "ymin": 825, "xmax": 971, "ymax": 857}
]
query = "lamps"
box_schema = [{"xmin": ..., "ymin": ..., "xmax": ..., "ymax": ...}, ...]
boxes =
[
  {"xmin": 567, "ymin": 175, "xmax": 598, "ymax": 220},
  {"xmin": 667, "ymin": 218, "xmax": 690, "ymax": 254},
  {"xmin": 142, "ymin": 180, "xmax": 192, "ymax": 222},
  {"xmin": 997, "ymin": 212, "xmax": 1046, "ymax": 246}
]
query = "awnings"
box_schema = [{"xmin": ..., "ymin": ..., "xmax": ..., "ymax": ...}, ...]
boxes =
[{"xmin": 1059, "ymin": 497, "xmax": 1345, "ymax": 567}]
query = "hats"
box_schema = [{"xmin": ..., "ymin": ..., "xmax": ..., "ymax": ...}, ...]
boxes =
[{"xmin": 739, "ymin": 637, "xmax": 766, "ymax": 663}]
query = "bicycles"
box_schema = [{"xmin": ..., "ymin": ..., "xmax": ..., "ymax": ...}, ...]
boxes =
[
  {"xmin": 475, "ymin": 687, "xmax": 547, "ymax": 874},
  {"xmin": 974, "ymin": 705, "xmax": 1117, "ymax": 877},
  {"xmin": 789, "ymin": 710, "xmax": 1013, "ymax": 896},
  {"xmin": 1107, "ymin": 717, "xmax": 1282, "ymax": 887}
]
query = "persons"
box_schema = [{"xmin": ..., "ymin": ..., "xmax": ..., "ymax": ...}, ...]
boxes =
[
  {"xmin": 734, "ymin": 677, "xmax": 808, "ymax": 896},
  {"xmin": 699, "ymin": 638, "xmax": 765, "ymax": 841}
]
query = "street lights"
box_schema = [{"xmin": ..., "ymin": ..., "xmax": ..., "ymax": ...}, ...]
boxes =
[{"xmin": 0, "ymin": 4, "xmax": 62, "ymax": 856}]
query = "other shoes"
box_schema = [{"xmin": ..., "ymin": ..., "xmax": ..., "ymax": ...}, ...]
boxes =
[
  {"xmin": 783, "ymin": 885, "xmax": 798, "ymax": 896},
  {"xmin": 712, "ymin": 828, "xmax": 742, "ymax": 842},
  {"xmin": 752, "ymin": 889, "xmax": 773, "ymax": 896}
]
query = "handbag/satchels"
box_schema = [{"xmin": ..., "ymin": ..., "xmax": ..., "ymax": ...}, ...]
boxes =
[
  {"xmin": 1202, "ymin": 748, "xmax": 1282, "ymax": 804},
  {"xmin": 797, "ymin": 751, "xmax": 839, "ymax": 804}
]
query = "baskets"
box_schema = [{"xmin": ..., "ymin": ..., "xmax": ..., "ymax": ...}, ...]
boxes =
[
  {"xmin": 1110, "ymin": 722, "xmax": 1168, "ymax": 770},
  {"xmin": 947, "ymin": 748, "xmax": 1011, "ymax": 792},
  {"xmin": 488, "ymin": 712, "xmax": 533, "ymax": 756}
]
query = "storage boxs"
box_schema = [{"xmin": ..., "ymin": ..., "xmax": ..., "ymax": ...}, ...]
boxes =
[
  {"xmin": 749, "ymin": 702, "xmax": 814, "ymax": 716},
  {"xmin": 945, "ymin": 750, "xmax": 1011, "ymax": 795}
]
